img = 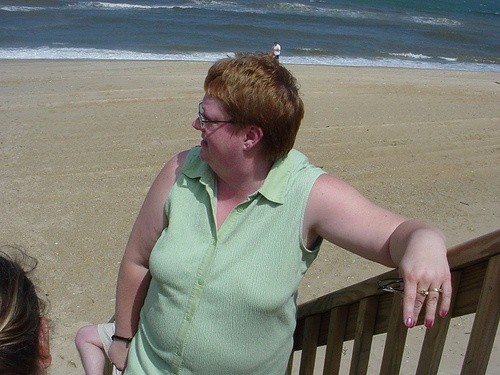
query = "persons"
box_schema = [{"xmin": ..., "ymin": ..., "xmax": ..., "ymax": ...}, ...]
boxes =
[
  {"xmin": 108, "ymin": 52, "xmax": 452, "ymax": 375},
  {"xmin": 75, "ymin": 322, "xmax": 115, "ymax": 375},
  {"xmin": 272, "ymin": 42, "xmax": 280, "ymax": 59},
  {"xmin": 0, "ymin": 257, "xmax": 52, "ymax": 375}
]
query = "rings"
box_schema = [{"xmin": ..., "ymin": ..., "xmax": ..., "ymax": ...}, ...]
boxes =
[
  {"xmin": 430, "ymin": 288, "xmax": 442, "ymax": 292},
  {"xmin": 417, "ymin": 289, "xmax": 428, "ymax": 295}
]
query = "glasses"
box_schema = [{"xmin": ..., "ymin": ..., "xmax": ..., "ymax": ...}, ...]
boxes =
[
  {"xmin": 198, "ymin": 101, "xmax": 250, "ymax": 130},
  {"xmin": 377, "ymin": 278, "xmax": 405, "ymax": 295}
]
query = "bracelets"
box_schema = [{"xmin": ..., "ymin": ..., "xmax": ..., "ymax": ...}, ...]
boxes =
[{"xmin": 112, "ymin": 335, "xmax": 132, "ymax": 342}]
